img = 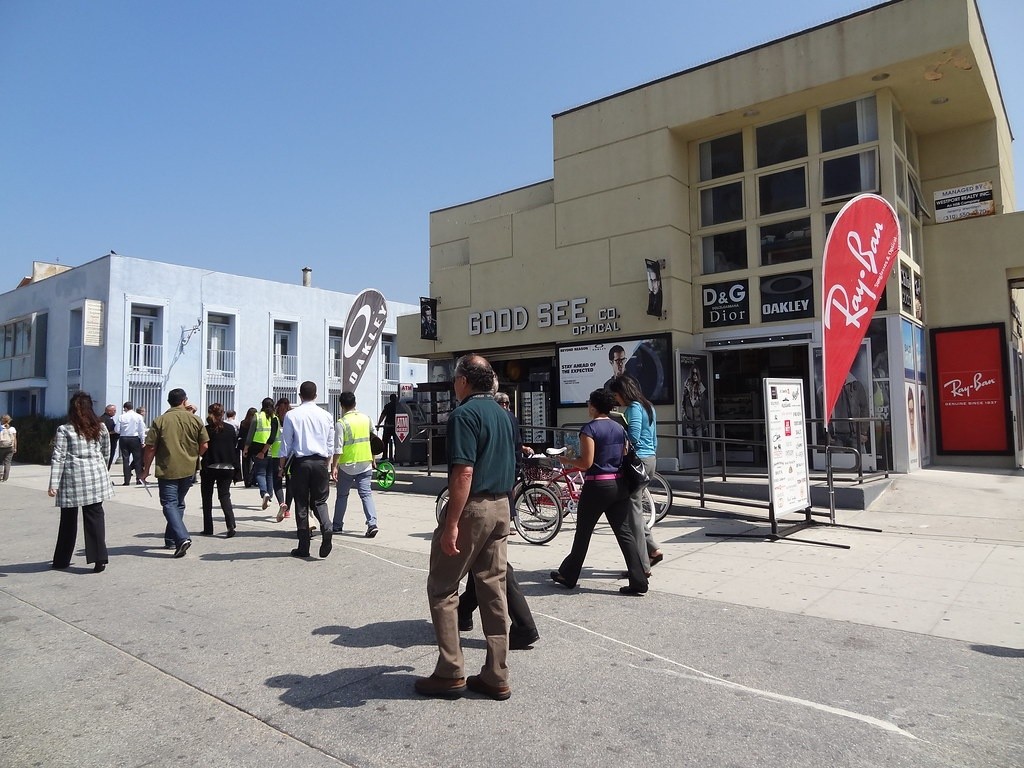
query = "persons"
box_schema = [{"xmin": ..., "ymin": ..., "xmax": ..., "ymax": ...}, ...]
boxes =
[
  {"xmin": 138, "ymin": 388, "xmax": 295, "ymax": 557},
  {"xmin": 98, "ymin": 402, "xmax": 148, "ymax": 487},
  {"xmin": 376, "ymin": 394, "xmax": 397, "ymax": 462},
  {"xmin": 816, "ymin": 372, "xmax": 867, "ymax": 454},
  {"xmin": 648, "ymin": 262, "xmax": 661, "ymax": 315},
  {"xmin": 432, "ymin": 361, "xmax": 450, "ymax": 421},
  {"xmin": 414, "ymin": 353, "xmax": 539, "ymax": 701},
  {"xmin": 331, "ymin": 391, "xmax": 378, "ymax": 538},
  {"xmin": 550, "ymin": 375, "xmax": 664, "ymax": 596},
  {"xmin": 907, "ymin": 386, "xmax": 931, "ymax": 458},
  {"xmin": 421, "ymin": 304, "xmax": 436, "ymax": 339},
  {"xmin": 604, "ymin": 345, "xmax": 642, "ymax": 399},
  {"xmin": 279, "ymin": 381, "xmax": 334, "ymax": 558},
  {"xmin": 48, "ymin": 391, "xmax": 111, "ymax": 573},
  {"xmin": 683, "ymin": 365, "xmax": 707, "ymax": 451},
  {"xmin": 0, "ymin": 415, "xmax": 17, "ymax": 482}
]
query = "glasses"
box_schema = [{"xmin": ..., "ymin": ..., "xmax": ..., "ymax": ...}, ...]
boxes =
[
  {"xmin": 497, "ymin": 401, "xmax": 510, "ymax": 406},
  {"xmin": 611, "ymin": 358, "xmax": 627, "ymax": 364},
  {"xmin": 451, "ymin": 375, "xmax": 465, "ymax": 384},
  {"xmin": 586, "ymin": 400, "xmax": 592, "ymax": 405}
]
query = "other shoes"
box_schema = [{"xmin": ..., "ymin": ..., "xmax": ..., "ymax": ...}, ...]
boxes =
[
  {"xmin": 365, "ymin": 525, "xmax": 378, "ymax": 537},
  {"xmin": 333, "ymin": 526, "xmax": 343, "ymax": 533},
  {"xmin": 261, "ymin": 493, "xmax": 290, "ymax": 522},
  {"xmin": 290, "ymin": 548, "xmax": 311, "ymax": 557},
  {"xmin": 319, "ymin": 529, "xmax": 333, "ymax": 558},
  {"xmin": 649, "ymin": 554, "xmax": 663, "ymax": 566},
  {"xmin": 620, "ymin": 584, "xmax": 648, "ymax": 594},
  {"xmin": 621, "ymin": 571, "xmax": 651, "ymax": 578},
  {"xmin": 94, "ymin": 563, "xmax": 105, "ymax": 573},
  {"xmin": 48, "ymin": 564, "xmax": 67, "ymax": 571},
  {"xmin": 509, "ymin": 624, "xmax": 540, "ymax": 647},
  {"xmin": 550, "ymin": 571, "xmax": 575, "ymax": 589},
  {"xmin": 162, "ymin": 527, "xmax": 237, "ymax": 558},
  {"xmin": 459, "ymin": 620, "xmax": 474, "ymax": 631}
]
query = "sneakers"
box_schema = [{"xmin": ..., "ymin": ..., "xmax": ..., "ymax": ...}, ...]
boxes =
[
  {"xmin": 467, "ymin": 675, "xmax": 511, "ymax": 700},
  {"xmin": 414, "ymin": 673, "xmax": 466, "ymax": 699}
]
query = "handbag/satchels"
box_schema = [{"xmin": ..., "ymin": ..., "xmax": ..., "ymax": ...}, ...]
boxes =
[{"xmin": 622, "ymin": 428, "xmax": 651, "ymax": 497}]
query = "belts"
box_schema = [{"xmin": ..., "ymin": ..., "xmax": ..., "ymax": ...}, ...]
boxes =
[{"xmin": 585, "ymin": 473, "xmax": 622, "ymax": 480}]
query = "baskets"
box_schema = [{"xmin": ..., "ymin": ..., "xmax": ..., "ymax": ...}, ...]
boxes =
[{"xmin": 520, "ymin": 458, "xmax": 554, "ymax": 480}]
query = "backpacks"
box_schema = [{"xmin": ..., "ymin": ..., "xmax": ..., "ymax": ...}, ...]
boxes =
[{"xmin": 0, "ymin": 426, "xmax": 13, "ymax": 449}]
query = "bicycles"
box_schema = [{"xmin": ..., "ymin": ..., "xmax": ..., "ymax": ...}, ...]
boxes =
[
  {"xmin": 435, "ymin": 447, "xmax": 673, "ymax": 544},
  {"xmin": 372, "ymin": 461, "xmax": 396, "ymax": 490}
]
